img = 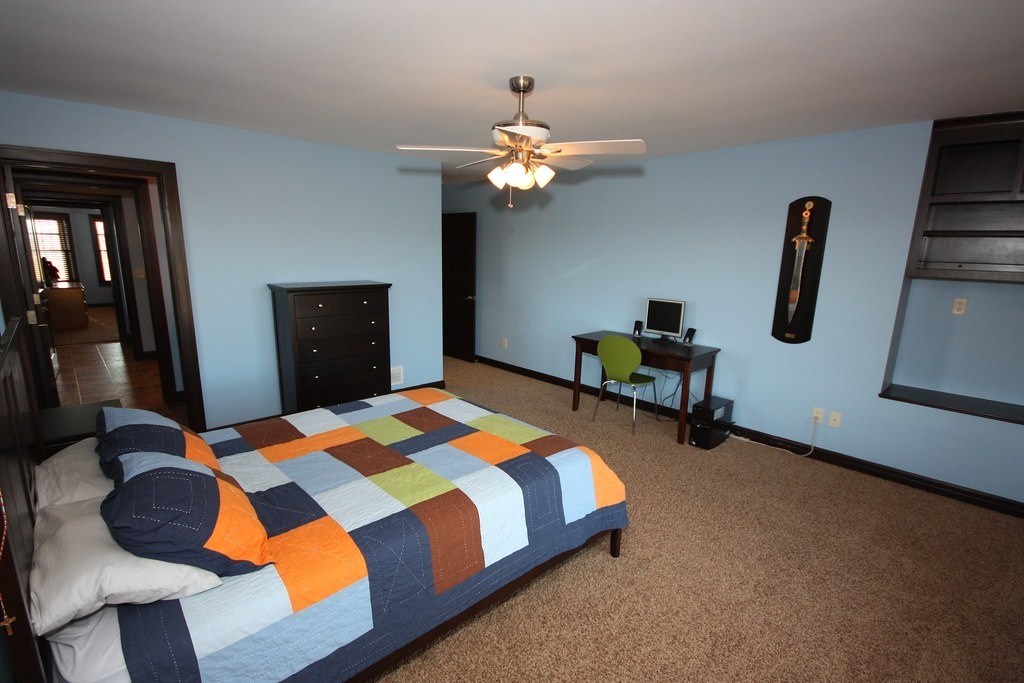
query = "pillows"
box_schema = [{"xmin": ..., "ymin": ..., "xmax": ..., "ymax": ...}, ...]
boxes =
[
  {"xmin": 96, "ymin": 407, "xmax": 277, "ymax": 576},
  {"xmin": 30, "ymin": 437, "xmax": 222, "ymax": 636}
]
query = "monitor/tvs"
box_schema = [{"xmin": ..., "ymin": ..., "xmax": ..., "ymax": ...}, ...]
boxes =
[{"xmin": 643, "ymin": 297, "xmax": 685, "ymax": 344}]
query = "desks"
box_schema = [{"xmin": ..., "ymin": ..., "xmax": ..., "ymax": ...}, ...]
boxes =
[{"xmin": 574, "ymin": 339, "xmax": 721, "ymax": 445}]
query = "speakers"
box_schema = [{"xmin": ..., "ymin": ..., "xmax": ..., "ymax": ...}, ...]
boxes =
[
  {"xmin": 683, "ymin": 328, "xmax": 696, "ymax": 347},
  {"xmin": 633, "ymin": 321, "xmax": 643, "ymax": 338}
]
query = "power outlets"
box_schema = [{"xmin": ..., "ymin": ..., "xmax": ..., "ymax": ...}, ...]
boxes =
[
  {"xmin": 829, "ymin": 411, "xmax": 841, "ymax": 428},
  {"xmin": 812, "ymin": 407, "xmax": 823, "ymax": 422}
]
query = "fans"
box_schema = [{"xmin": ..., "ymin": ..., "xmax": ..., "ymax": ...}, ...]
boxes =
[{"xmin": 396, "ymin": 76, "xmax": 646, "ymax": 171}]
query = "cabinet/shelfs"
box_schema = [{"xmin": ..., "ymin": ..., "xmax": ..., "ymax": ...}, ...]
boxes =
[
  {"xmin": 47, "ymin": 282, "xmax": 89, "ymax": 332},
  {"xmin": 267, "ymin": 280, "xmax": 392, "ymax": 416}
]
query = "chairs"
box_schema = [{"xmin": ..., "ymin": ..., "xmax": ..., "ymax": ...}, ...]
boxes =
[{"xmin": 591, "ymin": 335, "xmax": 659, "ymax": 435}]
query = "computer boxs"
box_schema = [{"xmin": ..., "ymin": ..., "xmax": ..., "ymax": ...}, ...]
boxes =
[{"xmin": 689, "ymin": 396, "xmax": 733, "ymax": 450}]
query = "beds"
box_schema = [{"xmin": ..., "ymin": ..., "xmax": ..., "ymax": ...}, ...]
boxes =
[{"xmin": 1, "ymin": 317, "xmax": 628, "ymax": 683}]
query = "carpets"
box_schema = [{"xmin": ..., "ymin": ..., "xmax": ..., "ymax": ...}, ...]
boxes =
[{"xmin": 35, "ymin": 399, "xmax": 123, "ymax": 441}]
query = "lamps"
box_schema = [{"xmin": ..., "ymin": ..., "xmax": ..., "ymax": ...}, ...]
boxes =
[{"xmin": 487, "ymin": 150, "xmax": 555, "ymax": 207}]
